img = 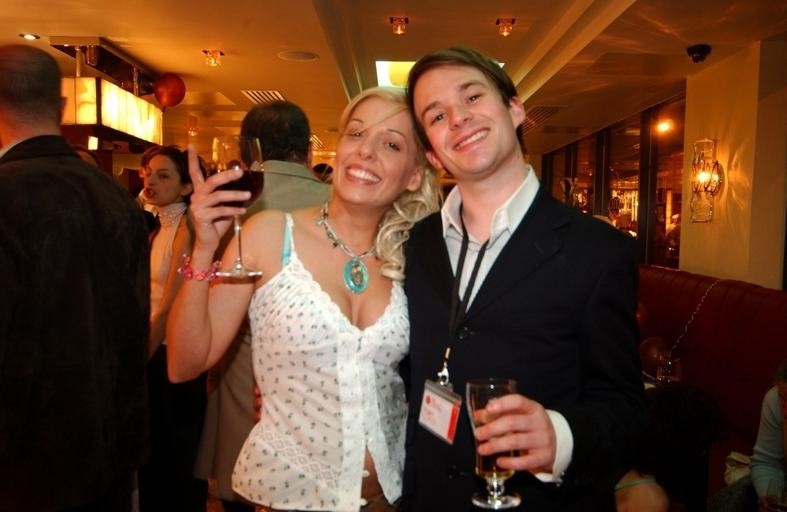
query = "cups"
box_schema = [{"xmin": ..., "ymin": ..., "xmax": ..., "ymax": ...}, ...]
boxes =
[{"xmin": 655, "ymin": 354, "xmax": 683, "ymax": 389}]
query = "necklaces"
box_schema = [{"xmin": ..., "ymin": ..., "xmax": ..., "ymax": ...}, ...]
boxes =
[{"xmin": 317, "ymin": 199, "xmax": 376, "ymax": 296}]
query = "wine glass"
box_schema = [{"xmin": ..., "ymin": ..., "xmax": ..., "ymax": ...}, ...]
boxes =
[
  {"xmin": 208, "ymin": 133, "xmax": 265, "ymax": 280},
  {"xmin": 464, "ymin": 376, "xmax": 520, "ymax": 510}
]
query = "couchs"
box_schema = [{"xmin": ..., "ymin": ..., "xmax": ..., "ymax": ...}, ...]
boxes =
[{"xmin": 637, "ymin": 264, "xmax": 787, "ymax": 512}]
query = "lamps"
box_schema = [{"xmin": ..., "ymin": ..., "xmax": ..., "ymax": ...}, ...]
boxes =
[
  {"xmin": 688, "ymin": 140, "xmax": 720, "ymax": 223},
  {"xmin": 206, "ymin": 51, "xmax": 220, "ymax": 67},
  {"xmin": 392, "ymin": 18, "xmax": 405, "ymax": 36},
  {"xmin": 499, "ymin": 18, "xmax": 514, "ymax": 37}
]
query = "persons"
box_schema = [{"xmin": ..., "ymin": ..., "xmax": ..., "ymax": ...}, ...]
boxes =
[
  {"xmin": 164, "ymin": 87, "xmax": 444, "ymax": 512},
  {"xmin": 709, "ymin": 369, "xmax": 787, "ymax": 510},
  {"xmin": 2, "ymin": 44, "xmax": 151, "ymax": 512},
  {"xmin": 186, "ymin": 97, "xmax": 334, "ymax": 511},
  {"xmin": 252, "ymin": 46, "xmax": 645, "ymax": 512},
  {"xmin": 77, "ymin": 144, "xmax": 332, "ymax": 509}
]
